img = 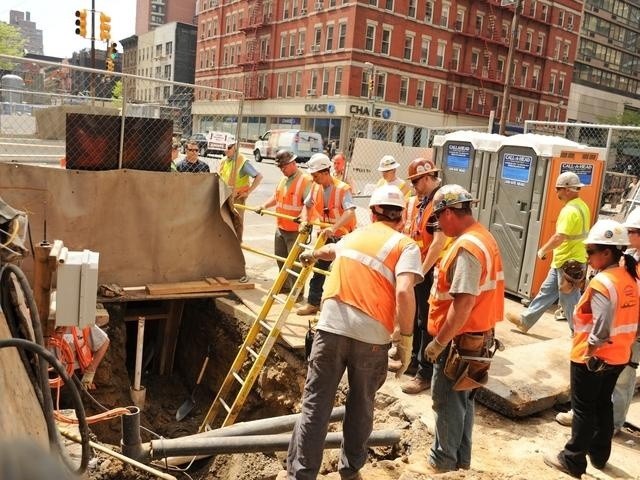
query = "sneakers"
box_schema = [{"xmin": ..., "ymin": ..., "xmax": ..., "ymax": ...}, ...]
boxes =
[
  {"xmin": 406, "ymin": 454, "xmax": 472, "ymax": 475},
  {"xmin": 401, "ymin": 376, "xmax": 432, "ymax": 394},
  {"xmin": 505, "ymin": 311, "xmax": 530, "ymax": 335},
  {"xmin": 338, "ymin": 469, "xmax": 363, "ymax": 480},
  {"xmin": 295, "ymin": 293, "xmax": 305, "ymax": 302},
  {"xmin": 553, "ymin": 408, "xmax": 573, "ymax": 426},
  {"xmin": 539, "ymin": 450, "xmax": 582, "ymax": 479},
  {"xmin": 277, "ymin": 286, "xmax": 292, "ymax": 295},
  {"xmin": 387, "ymin": 346, "xmax": 399, "ymax": 358},
  {"xmin": 296, "ymin": 304, "xmax": 320, "ymax": 316}
]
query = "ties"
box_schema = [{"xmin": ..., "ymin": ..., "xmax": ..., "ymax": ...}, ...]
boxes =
[{"xmin": 415, "ymin": 200, "xmax": 426, "ymax": 223}]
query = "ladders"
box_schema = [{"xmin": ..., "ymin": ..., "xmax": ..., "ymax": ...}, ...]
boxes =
[{"xmin": 195, "ymin": 226, "xmax": 329, "ymax": 433}]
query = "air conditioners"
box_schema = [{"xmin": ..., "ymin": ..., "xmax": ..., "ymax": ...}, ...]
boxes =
[
  {"xmin": 416, "ymin": 99, "xmax": 422, "ymax": 107},
  {"xmin": 296, "ymin": 49, "xmax": 303, "ymax": 55},
  {"xmin": 420, "ymin": 58, "xmax": 427, "ymax": 64},
  {"xmin": 209, "ymin": 62, "xmax": 213, "ymax": 67},
  {"xmin": 567, "ymin": 23, "xmax": 573, "ymax": 29},
  {"xmin": 310, "ymin": 45, "xmax": 319, "ymax": 51},
  {"xmin": 299, "ymin": 9, "xmax": 308, "ymax": 15},
  {"xmin": 201, "ymin": 34, "xmax": 204, "ymax": 39},
  {"xmin": 314, "ymin": 2, "xmax": 324, "ymax": 9},
  {"xmin": 426, "ymin": 17, "xmax": 431, "ymax": 23},
  {"xmin": 563, "ymin": 56, "xmax": 568, "ymax": 61},
  {"xmin": 307, "ymin": 89, "xmax": 315, "ymax": 96}
]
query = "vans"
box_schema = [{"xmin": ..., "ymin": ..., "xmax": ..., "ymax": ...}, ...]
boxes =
[{"xmin": 253, "ymin": 128, "xmax": 324, "ymax": 167}]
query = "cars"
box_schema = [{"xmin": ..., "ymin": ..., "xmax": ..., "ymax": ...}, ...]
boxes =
[{"xmin": 179, "ymin": 131, "xmax": 235, "ymax": 158}]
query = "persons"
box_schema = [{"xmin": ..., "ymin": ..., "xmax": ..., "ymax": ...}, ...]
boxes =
[
  {"xmin": 257, "ymin": 140, "xmax": 357, "ymax": 314},
  {"xmin": 408, "ymin": 183, "xmax": 508, "ymax": 477},
  {"xmin": 502, "ymin": 171, "xmax": 592, "ymax": 334},
  {"xmin": 275, "ymin": 185, "xmax": 426, "ymax": 480},
  {"xmin": 552, "ymin": 206, "xmax": 638, "ymax": 436},
  {"xmin": 47, "ymin": 291, "xmax": 112, "ymax": 409},
  {"xmin": 542, "ymin": 219, "xmax": 639, "ymax": 480},
  {"xmin": 171, "ymin": 137, "xmax": 264, "ymax": 241},
  {"xmin": 380, "ymin": 155, "xmax": 454, "ymax": 396}
]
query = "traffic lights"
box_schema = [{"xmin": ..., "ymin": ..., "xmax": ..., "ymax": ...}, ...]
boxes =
[
  {"xmin": 75, "ymin": 9, "xmax": 87, "ymax": 37},
  {"xmin": 101, "ymin": 13, "xmax": 111, "ymax": 41},
  {"xmin": 110, "ymin": 41, "xmax": 118, "ymax": 60},
  {"xmin": 369, "ymin": 80, "xmax": 375, "ymax": 91},
  {"xmin": 111, "ymin": 63, "xmax": 115, "ymax": 75},
  {"xmin": 105, "ymin": 60, "xmax": 111, "ymax": 77}
]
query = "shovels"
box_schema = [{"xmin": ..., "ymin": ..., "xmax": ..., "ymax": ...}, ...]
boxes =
[{"xmin": 176, "ymin": 343, "xmax": 215, "ymax": 422}]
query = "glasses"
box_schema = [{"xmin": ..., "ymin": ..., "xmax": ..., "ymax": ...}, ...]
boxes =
[
  {"xmin": 585, "ymin": 249, "xmax": 602, "ymax": 255},
  {"xmin": 228, "ymin": 144, "xmax": 234, "ymax": 151},
  {"xmin": 186, "ymin": 148, "xmax": 199, "ymax": 154},
  {"xmin": 435, "ymin": 209, "xmax": 447, "ymax": 219},
  {"xmin": 410, "ymin": 176, "xmax": 426, "ymax": 184}
]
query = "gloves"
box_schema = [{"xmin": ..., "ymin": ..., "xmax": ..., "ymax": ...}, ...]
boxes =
[
  {"xmin": 321, "ymin": 225, "xmax": 336, "ymax": 238},
  {"xmin": 387, "ymin": 345, "xmax": 413, "ymax": 380},
  {"xmin": 423, "ymin": 337, "xmax": 446, "ymax": 363},
  {"xmin": 253, "ymin": 204, "xmax": 265, "ymax": 215},
  {"xmin": 297, "ymin": 221, "xmax": 310, "ymax": 232},
  {"xmin": 79, "ymin": 369, "xmax": 96, "ymax": 391},
  {"xmin": 297, "ymin": 248, "xmax": 318, "ymax": 268},
  {"xmin": 536, "ymin": 247, "xmax": 547, "ymax": 259}
]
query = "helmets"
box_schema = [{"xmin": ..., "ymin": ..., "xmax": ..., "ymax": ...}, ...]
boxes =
[
  {"xmin": 375, "ymin": 154, "xmax": 401, "ymax": 173},
  {"xmin": 305, "ymin": 152, "xmax": 334, "ymax": 175},
  {"xmin": 581, "ymin": 218, "xmax": 633, "ymax": 248},
  {"xmin": 225, "ymin": 134, "xmax": 237, "ymax": 146},
  {"xmin": 172, "ymin": 135, "xmax": 183, "ymax": 148},
  {"xmin": 367, "ymin": 184, "xmax": 409, "ymax": 212},
  {"xmin": 623, "ymin": 206, "xmax": 640, "ymax": 231},
  {"xmin": 431, "ymin": 184, "xmax": 478, "ymax": 214},
  {"xmin": 404, "ymin": 157, "xmax": 442, "ymax": 184},
  {"xmin": 554, "ymin": 171, "xmax": 583, "ymax": 189},
  {"xmin": 273, "ymin": 148, "xmax": 299, "ymax": 166}
]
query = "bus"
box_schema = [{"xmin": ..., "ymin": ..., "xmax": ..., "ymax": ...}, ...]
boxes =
[{"xmin": 121, "ymin": 102, "xmax": 183, "ymax": 150}]
